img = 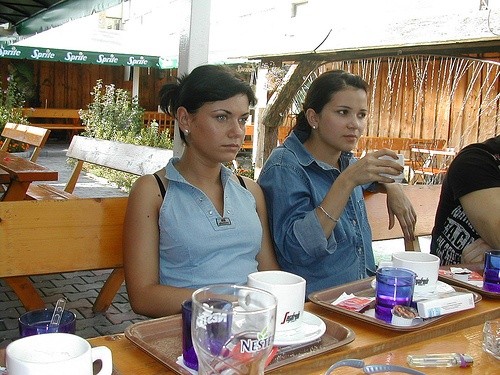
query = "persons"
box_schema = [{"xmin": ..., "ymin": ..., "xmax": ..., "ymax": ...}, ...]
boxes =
[
  {"xmin": 122, "ymin": 64, "xmax": 281, "ymax": 319},
  {"xmin": 254, "ymin": 66, "xmax": 417, "ymax": 306},
  {"xmin": 429, "ymin": 133, "xmax": 500, "ymax": 265}
]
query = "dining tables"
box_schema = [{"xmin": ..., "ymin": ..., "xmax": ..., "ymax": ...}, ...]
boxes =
[
  {"xmin": 0, "ymin": 263, "xmax": 500, "ymax": 375},
  {"xmin": 0, "ymin": 146, "xmax": 59, "ymax": 311}
]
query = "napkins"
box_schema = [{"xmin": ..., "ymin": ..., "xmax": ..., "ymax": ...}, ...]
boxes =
[{"xmin": 174, "ymin": 347, "xmax": 265, "ymax": 375}]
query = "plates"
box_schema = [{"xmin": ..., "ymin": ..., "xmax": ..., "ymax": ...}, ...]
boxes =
[
  {"xmin": 371, "ymin": 278, "xmax": 455, "ymax": 303},
  {"xmin": 231, "ymin": 306, "xmax": 327, "ymax": 347}
]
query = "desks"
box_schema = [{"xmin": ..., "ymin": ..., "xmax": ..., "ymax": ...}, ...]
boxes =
[{"xmin": 415, "ymin": 148, "xmax": 457, "ymax": 157}]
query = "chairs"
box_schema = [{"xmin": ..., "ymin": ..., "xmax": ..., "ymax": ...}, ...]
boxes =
[{"xmin": 414, "ymin": 155, "xmax": 439, "ymax": 187}]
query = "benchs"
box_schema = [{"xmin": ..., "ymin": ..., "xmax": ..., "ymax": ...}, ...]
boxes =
[
  {"xmin": 0, "ymin": 120, "xmax": 52, "ymax": 162},
  {"xmin": 26, "ymin": 134, "xmax": 174, "ymax": 199},
  {"xmin": 16, "ymin": 108, "xmax": 168, "ymax": 141},
  {"xmin": 355, "ymin": 139, "xmax": 449, "ymax": 182},
  {"xmin": 242, "ymin": 125, "xmax": 287, "ymax": 148},
  {"xmin": 0, "ymin": 161, "xmax": 476, "ymax": 282}
]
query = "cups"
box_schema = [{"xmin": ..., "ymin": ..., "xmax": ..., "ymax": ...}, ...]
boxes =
[
  {"xmin": 375, "ymin": 268, "xmax": 419, "ymax": 323},
  {"xmin": 482, "ymin": 249, "xmax": 500, "ymax": 294},
  {"xmin": 190, "ymin": 284, "xmax": 278, "ymax": 375},
  {"xmin": 377, "ymin": 251, "xmax": 440, "ymax": 294},
  {"xmin": 445, "ymin": 148, "xmax": 455, "ymax": 152},
  {"xmin": 180, "ymin": 298, "xmax": 234, "ymax": 371},
  {"xmin": 5, "ymin": 333, "xmax": 113, "ymax": 375},
  {"xmin": 238, "ymin": 270, "xmax": 307, "ymax": 332},
  {"xmin": 17, "ymin": 308, "xmax": 77, "ymax": 335},
  {"xmin": 378, "ymin": 154, "xmax": 405, "ymax": 184}
]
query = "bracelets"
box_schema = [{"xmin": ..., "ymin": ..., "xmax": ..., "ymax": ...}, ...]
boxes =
[{"xmin": 317, "ymin": 203, "xmax": 339, "ymax": 223}]
